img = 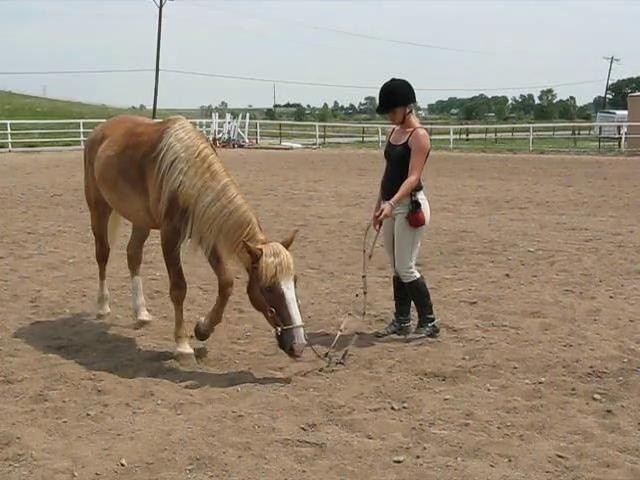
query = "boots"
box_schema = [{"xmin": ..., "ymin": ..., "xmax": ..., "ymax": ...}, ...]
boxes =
[{"xmin": 374, "ymin": 274, "xmax": 411, "ymax": 338}]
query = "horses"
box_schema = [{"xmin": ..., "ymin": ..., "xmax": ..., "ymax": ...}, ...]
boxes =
[{"xmin": 83, "ymin": 114, "xmax": 307, "ymax": 370}]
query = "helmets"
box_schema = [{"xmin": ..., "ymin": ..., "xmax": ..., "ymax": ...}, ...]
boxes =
[{"xmin": 376, "ymin": 79, "xmax": 416, "ymax": 114}]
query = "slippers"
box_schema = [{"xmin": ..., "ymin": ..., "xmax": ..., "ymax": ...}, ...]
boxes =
[{"xmin": 402, "ymin": 274, "xmax": 440, "ymax": 342}]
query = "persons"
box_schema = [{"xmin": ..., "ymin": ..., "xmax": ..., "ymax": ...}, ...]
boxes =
[{"xmin": 369, "ymin": 76, "xmax": 439, "ymax": 338}]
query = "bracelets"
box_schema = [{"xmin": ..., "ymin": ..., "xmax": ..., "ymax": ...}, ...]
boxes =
[{"xmin": 389, "ymin": 199, "xmax": 396, "ymax": 208}]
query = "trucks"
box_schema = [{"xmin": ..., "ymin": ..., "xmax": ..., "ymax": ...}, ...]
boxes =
[{"xmin": 595, "ymin": 108, "xmax": 628, "ymax": 136}]
